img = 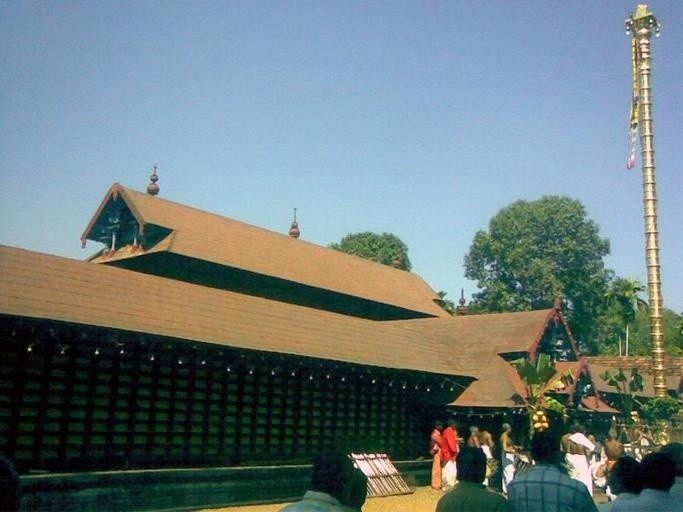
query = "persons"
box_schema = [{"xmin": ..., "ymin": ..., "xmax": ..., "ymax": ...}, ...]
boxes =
[
  {"xmin": 278, "ymin": 453, "xmax": 368, "ymax": 512},
  {"xmin": 430, "ymin": 419, "xmax": 682, "ymax": 512}
]
